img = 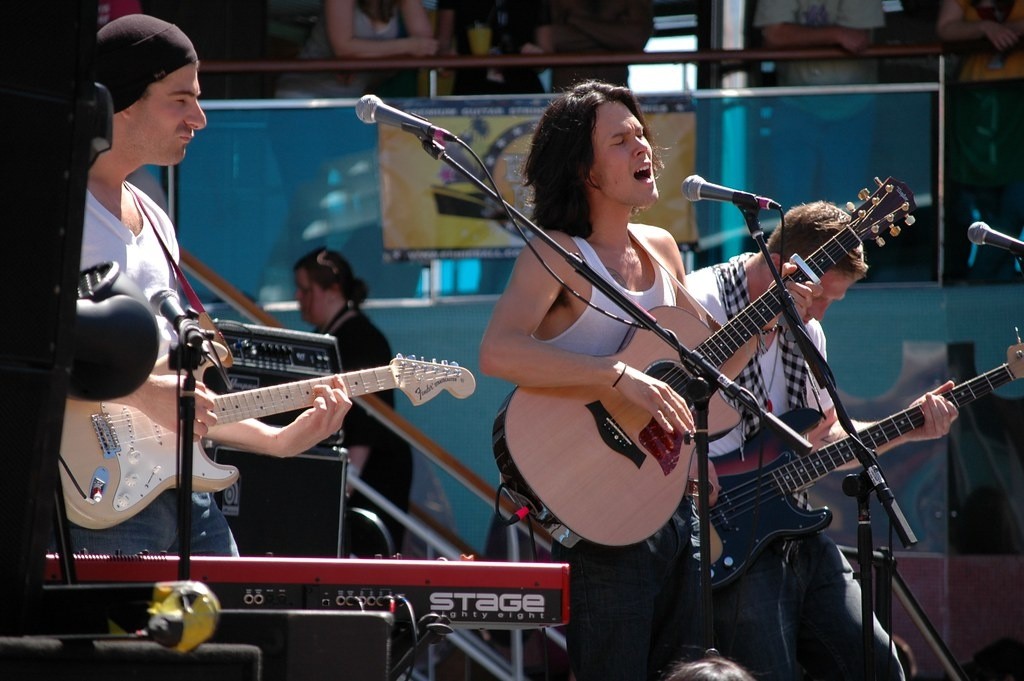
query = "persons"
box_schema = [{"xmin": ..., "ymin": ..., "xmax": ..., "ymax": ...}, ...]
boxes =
[
  {"xmin": 263, "ymin": 0, "xmax": 655, "ymax": 295},
  {"xmin": 45, "ymin": 13, "xmax": 353, "ymax": 558},
  {"xmin": 753, "ymin": 0, "xmax": 886, "ymax": 207},
  {"xmin": 674, "ymin": 199, "xmax": 959, "ymax": 681},
  {"xmin": 293, "ymin": 248, "xmax": 414, "ymax": 559},
  {"xmin": 666, "ymin": 656, "xmax": 755, "ymax": 681},
  {"xmin": 936, "ymin": 0, "xmax": 1024, "ymax": 283},
  {"xmin": 479, "ymin": 81, "xmax": 824, "ymax": 681}
]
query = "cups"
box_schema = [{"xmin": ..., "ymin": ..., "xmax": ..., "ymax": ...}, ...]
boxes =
[{"xmin": 469, "ymin": 23, "xmax": 490, "ymax": 55}]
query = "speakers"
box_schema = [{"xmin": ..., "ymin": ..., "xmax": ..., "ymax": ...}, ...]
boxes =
[
  {"xmin": 0, "ymin": 0, "xmax": 102, "ymax": 636},
  {"xmin": 203, "ymin": 438, "xmax": 349, "ymax": 562}
]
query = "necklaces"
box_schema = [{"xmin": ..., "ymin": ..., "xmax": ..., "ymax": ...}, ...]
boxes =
[{"xmin": 768, "ymin": 331, "xmax": 780, "ymax": 393}]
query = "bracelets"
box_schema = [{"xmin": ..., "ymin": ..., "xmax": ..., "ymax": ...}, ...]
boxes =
[
  {"xmin": 562, "ymin": 16, "xmax": 569, "ymax": 24},
  {"xmin": 762, "ymin": 321, "xmax": 778, "ymax": 335},
  {"xmin": 612, "ymin": 364, "xmax": 627, "ymax": 388}
]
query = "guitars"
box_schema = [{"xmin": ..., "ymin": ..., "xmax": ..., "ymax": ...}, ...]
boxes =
[
  {"xmin": 59, "ymin": 338, "xmax": 477, "ymax": 530},
  {"xmin": 697, "ymin": 326, "xmax": 1024, "ymax": 594},
  {"xmin": 492, "ymin": 175, "xmax": 917, "ymax": 559}
]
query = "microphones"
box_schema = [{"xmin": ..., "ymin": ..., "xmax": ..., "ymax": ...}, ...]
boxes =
[
  {"xmin": 149, "ymin": 288, "xmax": 203, "ymax": 348},
  {"xmin": 681, "ymin": 174, "xmax": 781, "ymax": 210},
  {"xmin": 356, "ymin": 95, "xmax": 457, "ymax": 143},
  {"xmin": 968, "ymin": 222, "xmax": 1024, "ymax": 255}
]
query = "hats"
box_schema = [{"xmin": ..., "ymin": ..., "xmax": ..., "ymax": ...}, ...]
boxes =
[{"xmin": 95, "ymin": 13, "xmax": 198, "ymax": 115}]
